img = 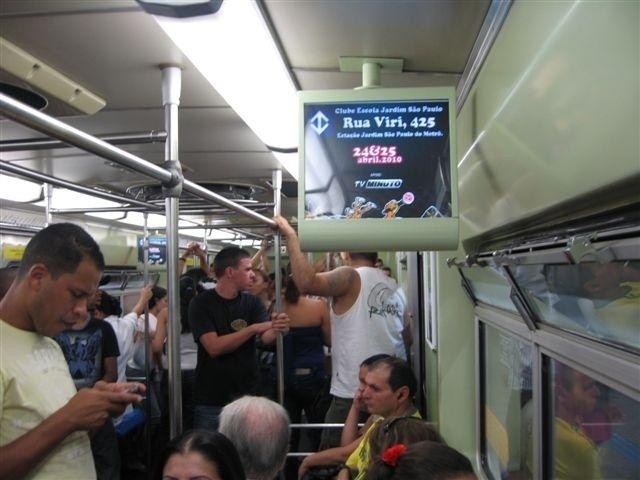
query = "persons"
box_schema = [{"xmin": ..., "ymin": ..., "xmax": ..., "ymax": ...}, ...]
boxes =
[
  {"xmin": 498, "ymin": 259, "xmax": 638, "ymax": 480},
  {"xmin": 0, "ymin": 216, "xmax": 482, "ymax": 480}
]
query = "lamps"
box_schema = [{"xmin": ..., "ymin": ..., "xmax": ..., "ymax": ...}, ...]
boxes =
[
  {"xmin": 136, "ymin": 0, "xmax": 301, "ymax": 182},
  {"xmin": 0, "ymin": 169, "xmax": 263, "ymax": 251}
]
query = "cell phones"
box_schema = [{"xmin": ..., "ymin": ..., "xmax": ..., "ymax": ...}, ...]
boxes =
[{"xmin": 124, "ymin": 383, "xmax": 139, "ymax": 394}]
